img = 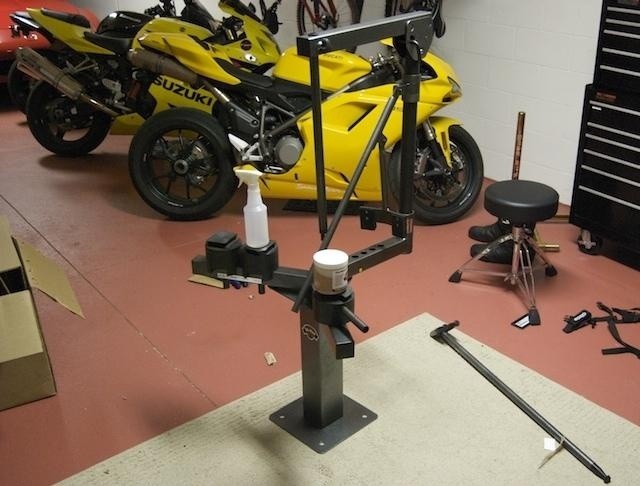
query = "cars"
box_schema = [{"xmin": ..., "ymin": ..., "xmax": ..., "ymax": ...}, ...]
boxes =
[{"xmin": 0, "ymin": 0, "xmax": 100, "ymax": 113}]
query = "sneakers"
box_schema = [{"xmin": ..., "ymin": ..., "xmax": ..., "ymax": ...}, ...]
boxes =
[
  {"xmin": 470, "ymin": 239, "xmax": 537, "ymax": 266},
  {"xmin": 469, "ymin": 219, "xmax": 536, "ymax": 242}
]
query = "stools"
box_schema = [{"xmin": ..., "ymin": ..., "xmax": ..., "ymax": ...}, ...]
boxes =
[{"xmin": 446, "ymin": 177, "xmax": 564, "ymax": 330}]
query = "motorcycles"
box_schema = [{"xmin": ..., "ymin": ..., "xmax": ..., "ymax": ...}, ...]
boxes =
[
  {"xmin": 84, "ymin": 0, "xmax": 483, "ymax": 225},
  {"xmin": 7, "ymin": 0, "xmax": 283, "ymax": 155}
]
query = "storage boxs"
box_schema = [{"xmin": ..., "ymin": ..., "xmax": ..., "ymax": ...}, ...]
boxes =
[{"xmin": 0, "ymin": 213, "xmax": 86, "ymax": 415}]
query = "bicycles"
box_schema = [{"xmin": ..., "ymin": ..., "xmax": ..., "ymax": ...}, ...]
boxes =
[{"xmin": 294, "ymin": 0, "xmax": 442, "ymax": 43}]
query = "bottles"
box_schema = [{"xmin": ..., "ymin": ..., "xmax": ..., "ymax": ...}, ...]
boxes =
[{"xmin": 312, "ymin": 247, "xmax": 348, "ymax": 295}]
